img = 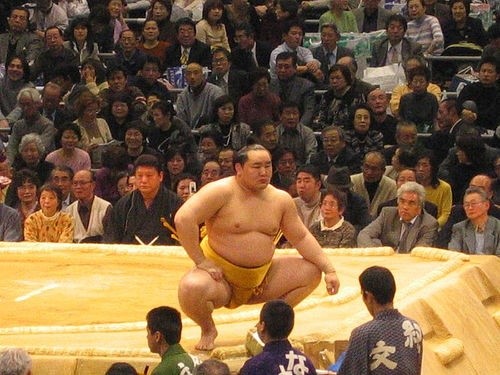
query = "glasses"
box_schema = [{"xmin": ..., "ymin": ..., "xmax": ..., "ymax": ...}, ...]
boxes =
[
  {"xmin": 82, "ymin": 106, "xmax": 101, "ymax": 116},
  {"xmin": 201, "ymin": 169, "xmax": 218, "ymax": 178},
  {"xmin": 463, "ymin": 202, "xmax": 484, "ymax": 209}
]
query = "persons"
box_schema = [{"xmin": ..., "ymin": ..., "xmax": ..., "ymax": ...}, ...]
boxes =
[
  {"xmin": 0, "ymin": 267, "xmax": 422, "ymax": 375},
  {"xmin": 0, "ymin": 0, "xmax": 500, "ymax": 255},
  {"xmin": 173, "ymin": 143, "xmax": 340, "ymax": 351}
]
